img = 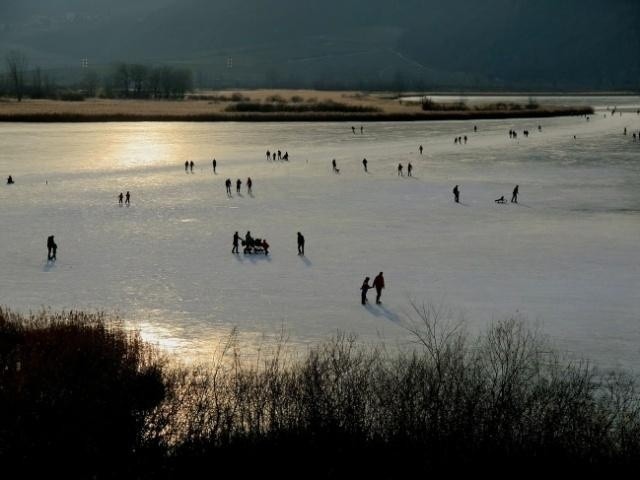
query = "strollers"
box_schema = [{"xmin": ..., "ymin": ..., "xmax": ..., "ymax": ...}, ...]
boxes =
[{"xmin": 242, "ymin": 239, "xmax": 265, "ymax": 254}]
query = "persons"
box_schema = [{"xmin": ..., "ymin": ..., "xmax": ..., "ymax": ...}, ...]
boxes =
[
  {"xmin": 296, "ymin": 232, "xmax": 305, "ymax": 255},
  {"xmin": 47, "ymin": 235, "xmax": 58, "ymax": 260},
  {"xmin": 473, "ymin": 125, "xmax": 478, "ymax": 132},
  {"xmin": 7, "ymin": 175, "xmax": 15, "ymax": 184},
  {"xmin": 509, "ymin": 125, "xmax": 542, "ymax": 137},
  {"xmin": 398, "ymin": 162, "xmax": 413, "ymax": 176},
  {"xmin": 118, "ymin": 190, "xmax": 130, "ymax": 204},
  {"xmin": 452, "ymin": 184, "xmax": 460, "ymax": 203},
  {"xmin": 212, "ymin": 158, "xmax": 217, "ymax": 171},
  {"xmin": 266, "ymin": 149, "xmax": 289, "ymax": 161},
  {"xmin": 183, "ymin": 159, "xmax": 194, "ymax": 171},
  {"xmin": 360, "ymin": 271, "xmax": 385, "ymax": 304},
  {"xmin": 577, "ymin": 105, "xmax": 623, "ymax": 121},
  {"xmin": 419, "ymin": 144, "xmax": 423, "ymax": 155},
  {"xmin": 352, "ymin": 125, "xmax": 364, "ymax": 134},
  {"xmin": 225, "ymin": 176, "xmax": 254, "ymax": 195},
  {"xmin": 454, "ymin": 135, "xmax": 468, "ymax": 144},
  {"xmin": 231, "ymin": 230, "xmax": 270, "ymax": 256},
  {"xmin": 622, "ymin": 127, "xmax": 637, "ymax": 141},
  {"xmin": 494, "ymin": 184, "xmax": 519, "ymax": 203},
  {"xmin": 332, "ymin": 158, "xmax": 340, "ymax": 173},
  {"xmin": 362, "ymin": 158, "xmax": 368, "ymax": 172}
]
